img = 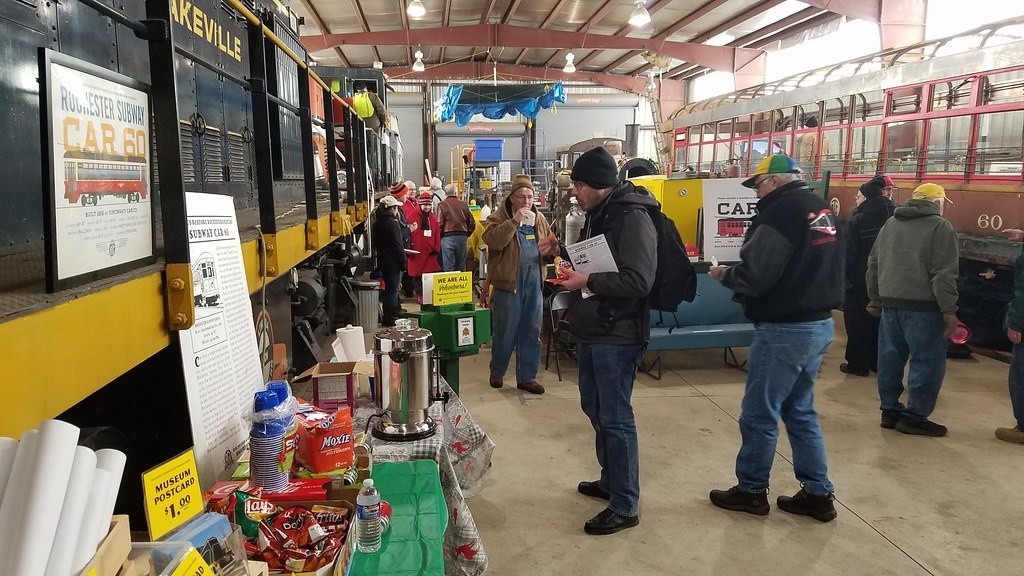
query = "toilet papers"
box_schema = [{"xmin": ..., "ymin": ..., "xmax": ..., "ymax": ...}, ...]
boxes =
[{"xmin": 336, "ymin": 326, "xmax": 372, "ymax": 397}]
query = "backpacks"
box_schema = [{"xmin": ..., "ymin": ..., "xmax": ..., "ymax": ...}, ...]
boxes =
[{"xmin": 615, "ymin": 204, "xmax": 698, "ymax": 313}]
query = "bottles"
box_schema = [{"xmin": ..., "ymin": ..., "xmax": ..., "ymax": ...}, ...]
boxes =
[{"xmin": 356, "ymin": 480, "xmax": 380, "ymax": 552}]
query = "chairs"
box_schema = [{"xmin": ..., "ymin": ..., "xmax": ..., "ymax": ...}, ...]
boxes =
[{"xmin": 546, "ymin": 290, "xmax": 577, "ymax": 381}]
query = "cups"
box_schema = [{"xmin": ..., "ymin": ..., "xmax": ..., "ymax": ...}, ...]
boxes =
[{"xmin": 252, "ymin": 380, "xmax": 295, "ymax": 493}]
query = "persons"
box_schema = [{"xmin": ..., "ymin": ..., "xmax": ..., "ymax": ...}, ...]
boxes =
[
  {"xmin": 995, "ymin": 228, "xmax": 1024, "ymax": 445},
  {"xmin": 838, "ymin": 176, "xmax": 901, "ymax": 378},
  {"xmin": 864, "ymin": 182, "xmax": 959, "ymax": 436},
  {"xmin": 400, "ymin": 181, "xmax": 418, "ymax": 298},
  {"xmin": 372, "ymin": 196, "xmax": 406, "ymax": 327},
  {"xmin": 797, "ymin": 132, "xmax": 830, "ymax": 162},
  {"xmin": 481, "ymin": 174, "xmax": 557, "ymax": 394},
  {"xmin": 437, "ymin": 184, "xmax": 475, "ymax": 272},
  {"xmin": 351, "ymin": 81, "xmax": 387, "ymax": 134},
  {"xmin": 406, "ymin": 192, "xmax": 441, "ymax": 305},
  {"xmin": 539, "ymin": 147, "xmax": 658, "ymax": 535},
  {"xmin": 709, "ymin": 154, "xmax": 838, "ymax": 523},
  {"xmin": 390, "ymin": 182, "xmax": 418, "ymax": 314},
  {"xmin": 430, "ymin": 177, "xmax": 446, "ymax": 216}
]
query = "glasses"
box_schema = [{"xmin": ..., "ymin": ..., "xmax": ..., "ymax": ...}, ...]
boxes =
[
  {"xmin": 512, "ymin": 193, "xmax": 534, "ymax": 200},
  {"xmin": 751, "ymin": 175, "xmax": 770, "ymax": 192},
  {"xmin": 573, "ymin": 181, "xmax": 587, "ymax": 189}
]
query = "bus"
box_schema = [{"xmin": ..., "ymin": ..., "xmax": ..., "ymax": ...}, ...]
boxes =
[{"xmin": 655, "ymin": 14, "xmax": 1024, "ymax": 268}]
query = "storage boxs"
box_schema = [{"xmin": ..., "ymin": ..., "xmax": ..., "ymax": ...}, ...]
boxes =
[
  {"xmin": 77, "ymin": 362, "xmax": 374, "ymax": 576},
  {"xmin": 473, "ymin": 138, "xmax": 506, "ymax": 160}
]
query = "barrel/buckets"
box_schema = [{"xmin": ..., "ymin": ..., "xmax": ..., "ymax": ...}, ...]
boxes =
[{"xmin": 372, "ymin": 326, "xmax": 449, "ymax": 441}]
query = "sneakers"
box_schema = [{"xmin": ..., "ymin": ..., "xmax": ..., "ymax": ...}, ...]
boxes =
[
  {"xmin": 490, "ymin": 371, "xmax": 503, "ymax": 388},
  {"xmin": 709, "ymin": 485, "xmax": 770, "ymax": 515},
  {"xmin": 894, "ymin": 415, "xmax": 947, "ymax": 437},
  {"xmin": 584, "ymin": 506, "xmax": 640, "ymax": 535},
  {"xmin": 578, "ymin": 480, "xmax": 608, "ymax": 500},
  {"xmin": 516, "ymin": 380, "xmax": 545, "ymax": 394},
  {"xmin": 776, "ymin": 481, "xmax": 837, "ymax": 522},
  {"xmin": 880, "ymin": 410, "xmax": 902, "ymax": 429},
  {"xmin": 995, "ymin": 426, "xmax": 1024, "ymax": 444}
]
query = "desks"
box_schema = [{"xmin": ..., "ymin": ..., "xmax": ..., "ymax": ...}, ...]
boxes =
[
  {"xmin": 351, "ymin": 459, "xmax": 450, "ymax": 576},
  {"xmin": 311, "ymin": 371, "xmax": 495, "ymax": 576}
]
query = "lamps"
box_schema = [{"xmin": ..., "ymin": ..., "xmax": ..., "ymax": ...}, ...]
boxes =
[
  {"xmin": 412, "ymin": 44, "xmax": 426, "ymax": 70},
  {"xmin": 563, "ymin": 49, "xmax": 576, "ymax": 73},
  {"xmin": 628, "ymin": 0, "xmax": 651, "ymax": 25},
  {"xmin": 406, "ymin": 0, "xmax": 426, "ymax": 17}
]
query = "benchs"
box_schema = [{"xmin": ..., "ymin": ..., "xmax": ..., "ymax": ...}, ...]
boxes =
[{"xmin": 640, "ymin": 274, "xmax": 756, "ymax": 380}]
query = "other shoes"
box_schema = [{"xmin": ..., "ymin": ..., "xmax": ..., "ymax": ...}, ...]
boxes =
[
  {"xmin": 382, "ymin": 319, "xmax": 396, "ymax": 326},
  {"xmin": 404, "ymin": 289, "xmax": 413, "ymax": 298},
  {"xmin": 840, "ymin": 362, "xmax": 869, "ymax": 376},
  {"xmin": 417, "ymin": 296, "xmax": 424, "ymax": 304},
  {"xmin": 396, "ymin": 308, "xmax": 407, "ymax": 318}
]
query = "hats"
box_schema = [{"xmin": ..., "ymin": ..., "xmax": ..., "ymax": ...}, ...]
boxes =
[
  {"xmin": 912, "ymin": 182, "xmax": 954, "ymax": 205},
  {"xmin": 570, "ymin": 146, "xmax": 616, "ymax": 189},
  {"xmin": 874, "ymin": 173, "xmax": 894, "ymax": 186},
  {"xmin": 379, "ymin": 196, "xmax": 403, "ymax": 208},
  {"xmin": 741, "ymin": 154, "xmax": 801, "ymax": 188},
  {"xmin": 510, "ymin": 174, "xmax": 535, "ymax": 196},
  {"xmin": 389, "ymin": 181, "xmax": 408, "ymax": 198},
  {"xmin": 418, "ymin": 192, "xmax": 432, "ymax": 205},
  {"xmin": 859, "ymin": 178, "xmax": 886, "ymax": 198}
]
